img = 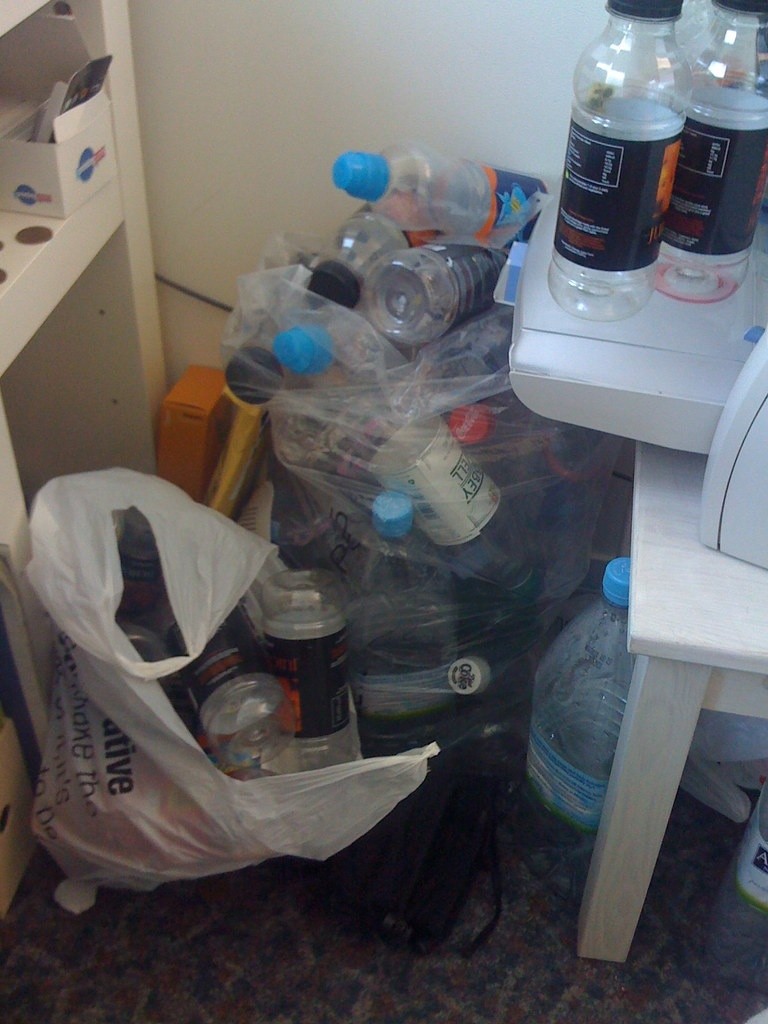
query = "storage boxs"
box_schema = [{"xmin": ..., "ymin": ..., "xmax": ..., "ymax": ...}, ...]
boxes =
[
  {"xmin": 156, "ymin": 365, "xmax": 230, "ymax": 503},
  {"xmin": 0, "ymin": 10, "xmax": 119, "ymax": 217},
  {"xmin": 0, "ymin": 717, "xmax": 39, "ymax": 917}
]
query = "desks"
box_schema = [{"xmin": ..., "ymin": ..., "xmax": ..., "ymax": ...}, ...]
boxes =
[{"xmin": 504, "ymin": 202, "xmax": 768, "ymax": 963}]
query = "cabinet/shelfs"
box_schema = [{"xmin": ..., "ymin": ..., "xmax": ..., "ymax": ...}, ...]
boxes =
[{"xmin": 0, "ymin": 0, "xmax": 167, "ymax": 791}]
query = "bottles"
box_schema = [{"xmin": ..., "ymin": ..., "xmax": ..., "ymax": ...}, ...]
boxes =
[
  {"xmin": 656, "ymin": 0, "xmax": 768, "ymax": 305},
  {"xmin": 106, "ymin": 151, "xmax": 639, "ymax": 896},
  {"xmin": 703, "ymin": 779, "xmax": 768, "ymax": 992},
  {"xmin": 549, "ymin": 0, "xmax": 688, "ymax": 324}
]
